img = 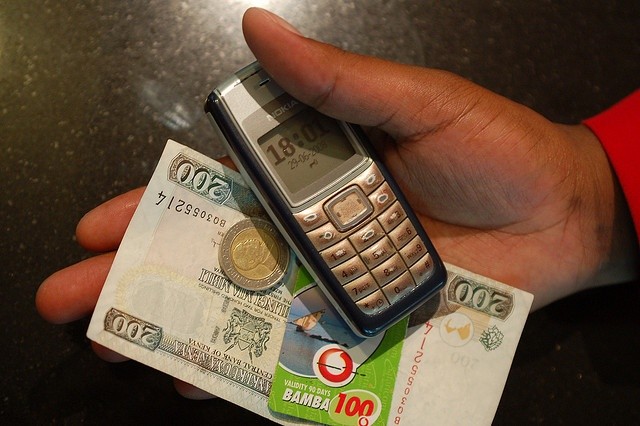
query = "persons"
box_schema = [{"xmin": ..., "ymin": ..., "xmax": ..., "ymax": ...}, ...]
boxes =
[{"xmin": 34, "ymin": 3, "xmax": 637, "ymax": 400}]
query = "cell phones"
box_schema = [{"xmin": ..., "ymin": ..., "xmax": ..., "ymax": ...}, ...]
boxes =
[{"xmin": 204, "ymin": 58, "xmax": 449, "ymax": 339}]
